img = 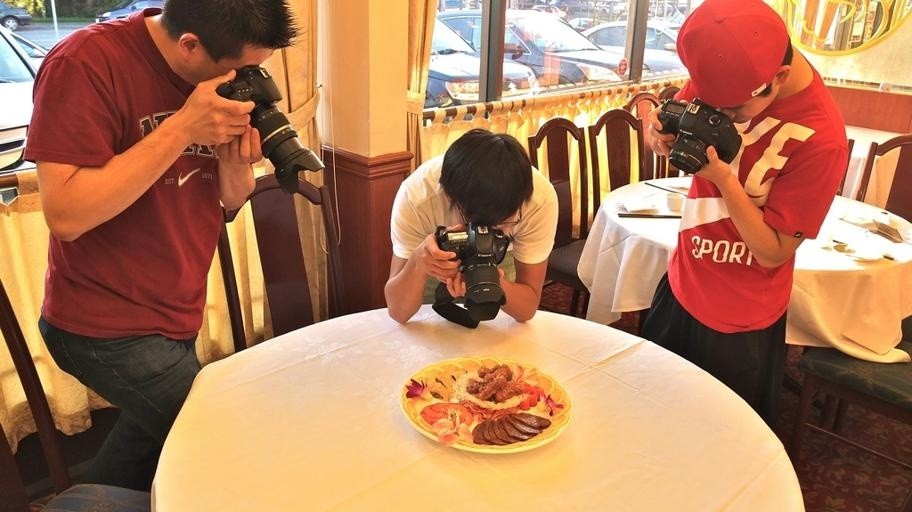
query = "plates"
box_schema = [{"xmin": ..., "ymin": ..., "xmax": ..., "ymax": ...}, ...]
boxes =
[{"xmin": 400, "ymin": 356, "xmax": 573, "ymax": 454}]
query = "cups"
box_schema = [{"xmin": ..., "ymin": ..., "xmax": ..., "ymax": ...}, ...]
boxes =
[{"xmin": 667, "ymin": 193, "xmax": 685, "ymax": 212}]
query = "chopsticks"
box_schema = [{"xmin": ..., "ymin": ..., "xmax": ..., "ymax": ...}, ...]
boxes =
[
  {"xmin": 833, "ymin": 240, "xmax": 893, "ymax": 261},
  {"xmin": 618, "ymin": 214, "xmax": 682, "ymax": 218},
  {"xmin": 645, "ymin": 182, "xmax": 687, "ymax": 197}
]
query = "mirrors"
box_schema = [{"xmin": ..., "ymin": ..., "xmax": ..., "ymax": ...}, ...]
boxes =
[{"xmin": 772, "ymin": 0, "xmax": 910, "ymax": 54}]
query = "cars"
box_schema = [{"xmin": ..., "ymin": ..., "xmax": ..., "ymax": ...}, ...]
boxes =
[{"xmin": 0, "ymin": 0, "xmax": 170, "ymax": 176}]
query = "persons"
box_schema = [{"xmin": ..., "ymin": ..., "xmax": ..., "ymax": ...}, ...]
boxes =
[
  {"xmin": 385, "ymin": 128, "xmax": 559, "ymax": 325},
  {"xmin": 638, "ymin": 1, "xmax": 848, "ymax": 434},
  {"xmin": 21, "ymin": 0, "xmax": 304, "ymax": 495}
]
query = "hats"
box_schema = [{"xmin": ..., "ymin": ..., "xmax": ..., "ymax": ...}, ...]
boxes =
[{"xmin": 673, "ymin": 0, "xmax": 790, "ymax": 109}]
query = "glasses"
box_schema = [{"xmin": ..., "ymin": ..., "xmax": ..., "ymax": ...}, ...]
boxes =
[{"xmin": 455, "ymin": 198, "xmax": 523, "ymax": 230}]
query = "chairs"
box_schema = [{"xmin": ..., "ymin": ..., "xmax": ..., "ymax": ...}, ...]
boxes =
[
  {"xmin": 792, "ymin": 331, "xmax": 912, "ymax": 463},
  {"xmin": 834, "ymin": 139, "xmax": 855, "ymax": 197},
  {"xmin": 216, "ymin": 173, "xmax": 346, "ymax": 352},
  {"xmin": 587, "ymin": 110, "xmax": 648, "ymax": 223},
  {"xmin": 525, "ymin": 117, "xmax": 606, "ymax": 320},
  {"xmin": 626, "ymin": 93, "xmax": 668, "ymax": 180},
  {"xmin": 855, "ymin": 132, "xmax": 911, "ymax": 220},
  {"xmin": 0, "ymin": 283, "xmax": 153, "ymax": 512},
  {"xmin": 660, "ymin": 85, "xmax": 689, "ymax": 176}
]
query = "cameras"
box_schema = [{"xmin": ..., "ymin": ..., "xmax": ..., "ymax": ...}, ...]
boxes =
[
  {"xmin": 435, "ymin": 223, "xmax": 510, "ymax": 322},
  {"xmin": 657, "ymin": 96, "xmax": 742, "ymax": 174},
  {"xmin": 217, "ymin": 63, "xmax": 324, "ymax": 194}
]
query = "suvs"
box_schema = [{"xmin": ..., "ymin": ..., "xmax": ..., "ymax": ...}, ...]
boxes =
[{"xmin": 426, "ymin": 10, "xmax": 684, "ymax": 109}]
